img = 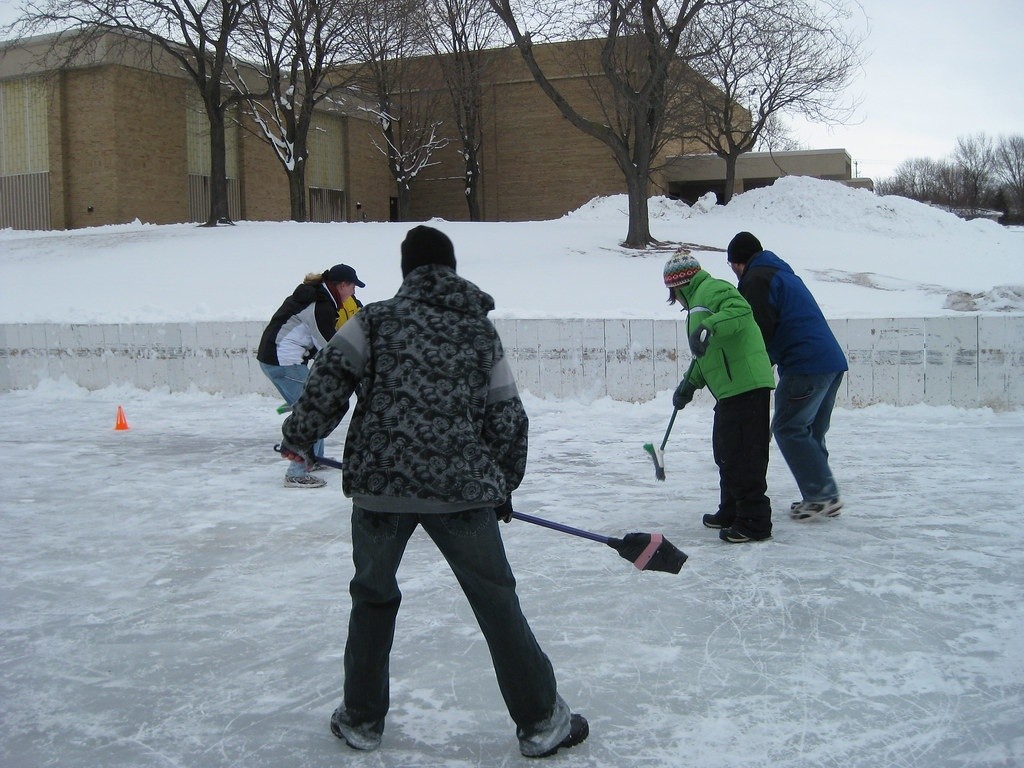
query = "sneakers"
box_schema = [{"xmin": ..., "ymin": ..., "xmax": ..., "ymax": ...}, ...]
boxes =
[
  {"xmin": 720, "ymin": 525, "xmax": 773, "ymax": 543},
  {"xmin": 702, "ymin": 509, "xmax": 723, "ymax": 528},
  {"xmin": 790, "ymin": 497, "xmax": 843, "ymax": 523}
]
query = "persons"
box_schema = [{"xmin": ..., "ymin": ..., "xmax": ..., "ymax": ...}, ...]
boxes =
[
  {"xmin": 279, "ymin": 224, "xmax": 589, "ymax": 758},
  {"xmin": 727, "ymin": 232, "xmax": 849, "ymax": 521},
  {"xmin": 663, "ymin": 243, "xmax": 776, "ymax": 543},
  {"xmin": 256, "ymin": 263, "xmax": 366, "ymax": 488}
]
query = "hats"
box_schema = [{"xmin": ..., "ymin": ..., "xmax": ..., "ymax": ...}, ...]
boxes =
[
  {"xmin": 331, "ymin": 263, "xmax": 366, "ymax": 288},
  {"xmin": 728, "ymin": 231, "xmax": 763, "ymax": 265},
  {"xmin": 663, "ymin": 245, "xmax": 701, "ymax": 287},
  {"xmin": 401, "ymin": 225, "xmax": 456, "ymax": 278}
]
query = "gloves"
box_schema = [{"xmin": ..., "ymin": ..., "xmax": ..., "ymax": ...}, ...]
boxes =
[
  {"xmin": 495, "ymin": 493, "xmax": 513, "ymax": 524},
  {"xmin": 688, "ymin": 324, "xmax": 710, "ymax": 359},
  {"xmin": 672, "ymin": 379, "xmax": 696, "ymax": 409}
]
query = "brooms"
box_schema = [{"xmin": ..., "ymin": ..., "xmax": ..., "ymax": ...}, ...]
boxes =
[
  {"xmin": 276, "ymin": 402, "xmax": 293, "ymax": 415},
  {"xmin": 643, "ymin": 329, "xmax": 708, "ymax": 481},
  {"xmin": 273, "ymin": 442, "xmax": 691, "ymax": 575}
]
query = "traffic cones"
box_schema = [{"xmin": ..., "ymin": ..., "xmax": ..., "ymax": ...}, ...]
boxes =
[{"xmin": 111, "ymin": 405, "xmax": 130, "ymax": 431}]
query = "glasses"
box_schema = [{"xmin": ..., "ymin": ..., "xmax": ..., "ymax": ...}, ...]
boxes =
[{"xmin": 728, "ymin": 262, "xmax": 736, "ymax": 268}]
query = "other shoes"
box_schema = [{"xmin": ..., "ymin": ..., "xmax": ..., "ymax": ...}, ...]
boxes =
[
  {"xmin": 329, "ymin": 713, "xmax": 366, "ymax": 750},
  {"xmin": 284, "ymin": 473, "xmax": 327, "ymax": 488},
  {"xmin": 522, "ymin": 712, "xmax": 591, "ymax": 759},
  {"xmin": 306, "ymin": 457, "xmax": 336, "ymax": 472}
]
